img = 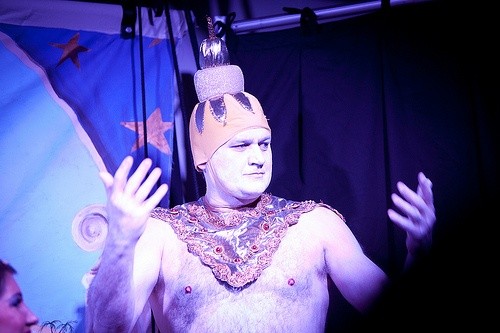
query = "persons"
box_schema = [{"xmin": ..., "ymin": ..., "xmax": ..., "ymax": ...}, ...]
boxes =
[
  {"xmin": 86, "ymin": 92, "xmax": 437, "ymax": 333},
  {"xmin": 0, "ymin": 258, "xmax": 39, "ymax": 333}
]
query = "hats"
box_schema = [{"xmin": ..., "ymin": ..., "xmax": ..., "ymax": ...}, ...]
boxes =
[{"xmin": 188, "ymin": 15, "xmax": 271, "ymax": 171}]
